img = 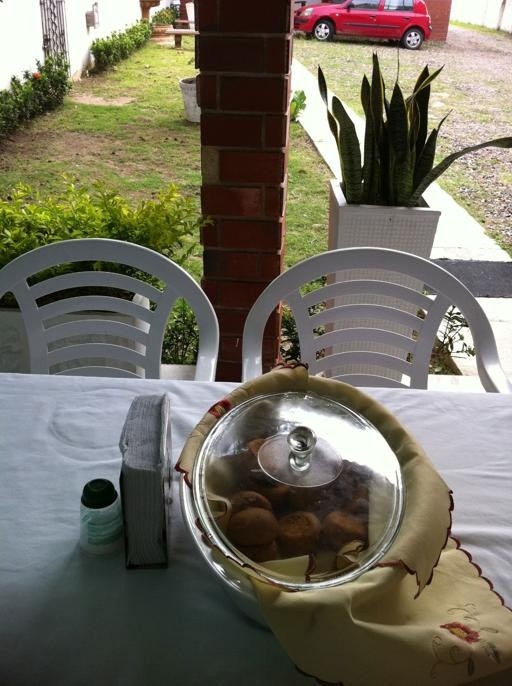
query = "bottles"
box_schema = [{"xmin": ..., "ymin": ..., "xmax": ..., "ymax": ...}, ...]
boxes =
[{"xmin": 79, "ymin": 479, "xmax": 121, "ymax": 557}]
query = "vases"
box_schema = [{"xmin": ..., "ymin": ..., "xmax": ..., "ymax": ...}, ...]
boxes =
[{"xmin": 178, "ymin": 76, "xmax": 201, "ymax": 123}]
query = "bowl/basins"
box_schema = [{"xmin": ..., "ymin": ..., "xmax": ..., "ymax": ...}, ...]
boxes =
[{"xmin": 182, "ymin": 374, "xmax": 429, "ymax": 607}]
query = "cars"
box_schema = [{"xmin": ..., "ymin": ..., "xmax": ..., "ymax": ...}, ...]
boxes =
[{"xmin": 293, "ymin": 0, "xmax": 431, "ymax": 48}]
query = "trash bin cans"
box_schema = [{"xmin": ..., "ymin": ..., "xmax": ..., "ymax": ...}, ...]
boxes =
[{"xmin": 171, "ymin": 4, "xmax": 180, "ymax": 19}]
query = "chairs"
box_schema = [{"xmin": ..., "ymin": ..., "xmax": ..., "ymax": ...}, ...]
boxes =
[
  {"xmin": 0, "ymin": 237, "xmax": 220, "ymax": 382},
  {"xmin": 241, "ymin": 245, "xmax": 511, "ymax": 394}
]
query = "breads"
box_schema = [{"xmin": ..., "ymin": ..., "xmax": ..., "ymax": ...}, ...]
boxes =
[{"xmin": 216, "ymin": 438, "xmax": 369, "ymax": 561}]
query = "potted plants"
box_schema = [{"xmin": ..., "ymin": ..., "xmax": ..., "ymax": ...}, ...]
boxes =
[{"xmin": 317, "ymin": 43, "xmax": 512, "ymax": 383}]
